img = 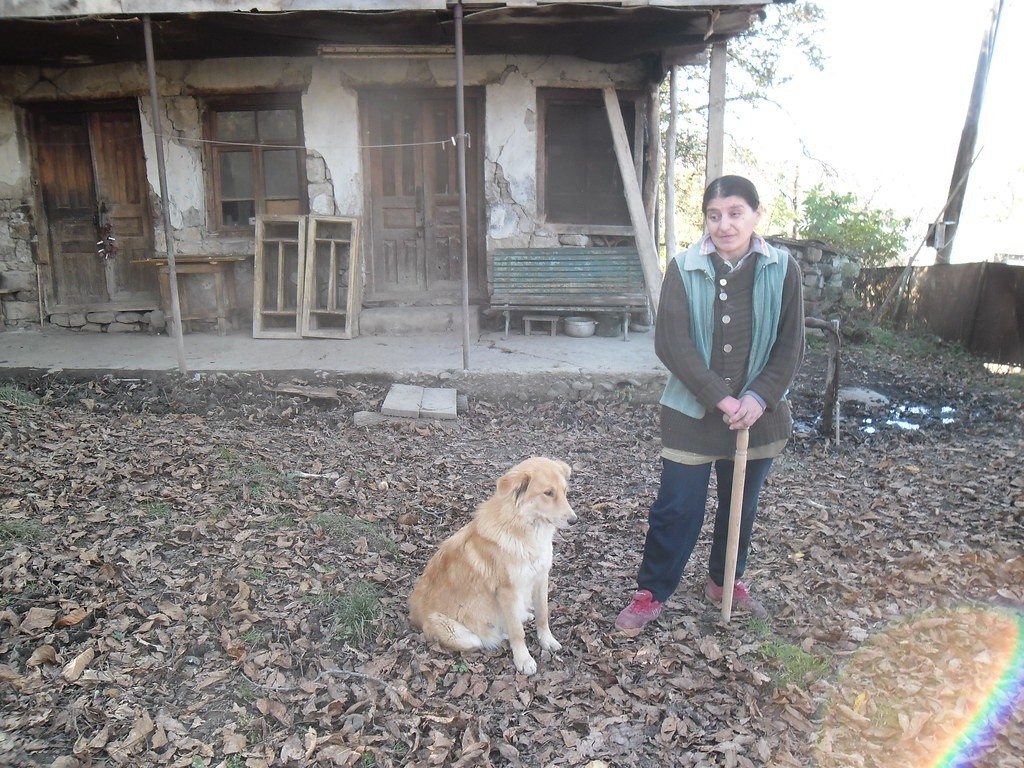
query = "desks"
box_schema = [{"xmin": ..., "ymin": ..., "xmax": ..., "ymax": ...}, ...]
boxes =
[{"xmin": 129, "ymin": 254, "xmax": 255, "ymax": 338}]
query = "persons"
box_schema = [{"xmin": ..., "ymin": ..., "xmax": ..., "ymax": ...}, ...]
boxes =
[{"xmin": 615, "ymin": 175, "xmax": 806, "ymax": 630}]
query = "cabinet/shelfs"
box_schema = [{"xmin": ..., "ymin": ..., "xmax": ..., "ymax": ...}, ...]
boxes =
[
  {"xmin": 253, "ymin": 214, "xmax": 310, "ymax": 340},
  {"xmin": 522, "ymin": 314, "xmax": 561, "ymax": 336},
  {"xmin": 302, "ymin": 215, "xmax": 362, "ymax": 339}
]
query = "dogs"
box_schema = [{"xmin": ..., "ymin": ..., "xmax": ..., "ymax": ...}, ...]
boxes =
[{"xmin": 407, "ymin": 457, "xmax": 578, "ymax": 674}]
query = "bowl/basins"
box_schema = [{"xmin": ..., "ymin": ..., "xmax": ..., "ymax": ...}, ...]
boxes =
[{"xmin": 563, "ymin": 316, "xmax": 597, "ymax": 337}]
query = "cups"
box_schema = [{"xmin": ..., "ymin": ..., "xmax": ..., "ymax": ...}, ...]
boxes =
[{"xmin": 249, "ymin": 218, "xmax": 256, "ymax": 226}]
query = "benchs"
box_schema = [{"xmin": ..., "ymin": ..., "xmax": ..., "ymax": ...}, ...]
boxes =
[{"xmin": 490, "ymin": 246, "xmax": 650, "ymax": 342}]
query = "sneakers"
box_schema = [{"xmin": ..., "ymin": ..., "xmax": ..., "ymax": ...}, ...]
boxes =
[
  {"xmin": 614, "ymin": 590, "xmax": 662, "ymax": 632},
  {"xmin": 704, "ymin": 575, "xmax": 771, "ymax": 625}
]
